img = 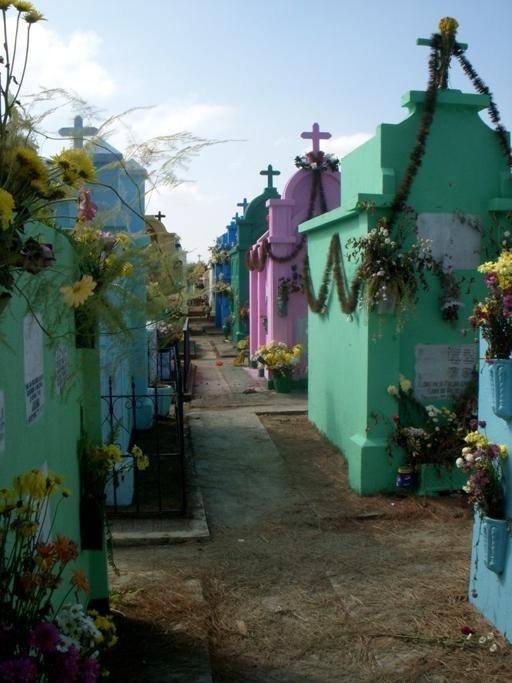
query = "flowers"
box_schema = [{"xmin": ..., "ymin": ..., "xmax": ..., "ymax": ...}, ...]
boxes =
[
  {"xmin": 1, "ymin": 1, "xmax": 249, "ymax": 356},
  {"xmin": 255, "ymin": 339, "xmax": 304, "ymax": 370},
  {"xmin": 455, "ymin": 418, "xmax": 509, "ymax": 519},
  {"xmin": 385, "ymin": 376, "xmax": 478, "ymax": 470},
  {"xmin": 1, "ymin": 430, "xmax": 155, "ymax": 682},
  {"xmin": 277, "ymin": 262, "xmax": 301, "ymax": 303},
  {"xmin": 467, "ymin": 247, "xmax": 512, "ymax": 364},
  {"xmin": 345, "ymin": 217, "xmax": 435, "ymax": 343}
]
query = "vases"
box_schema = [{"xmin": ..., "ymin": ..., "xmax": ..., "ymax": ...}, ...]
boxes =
[
  {"xmin": 479, "ymin": 515, "xmax": 506, "ymax": 577},
  {"xmin": 417, "ymin": 462, "xmax": 479, "ymax": 497},
  {"xmin": 377, "ymin": 281, "xmax": 398, "ymax": 316},
  {"xmin": 276, "ymin": 296, "xmax": 288, "ymax": 318},
  {"xmin": 489, "ymin": 359, "xmax": 512, "ymax": 420},
  {"xmin": 271, "ymin": 370, "xmax": 293, "ymax": 394}
]
